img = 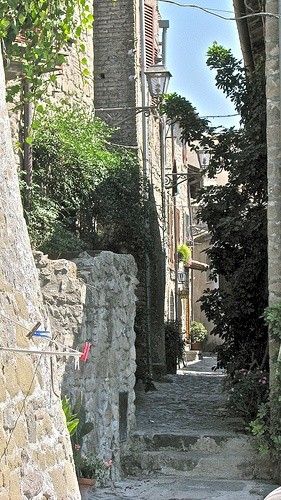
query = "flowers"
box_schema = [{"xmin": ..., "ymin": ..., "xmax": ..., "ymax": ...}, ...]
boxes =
[{"xmin": 73, "ymin": 444, "xmax": 113, "ymax": 479}]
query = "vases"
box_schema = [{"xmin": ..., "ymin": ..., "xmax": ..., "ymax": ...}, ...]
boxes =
[{"xmin": 77, "ymin": 477, "xmax": 97, "ymax": 500}]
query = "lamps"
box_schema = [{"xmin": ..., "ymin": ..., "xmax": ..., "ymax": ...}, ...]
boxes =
[
  {"xmin": 164, "ymin": 141, "xmax": 223, "ymax": 189},
  {"xmin": 92, "ymin": 48, "xmax": 173, "ymax": 133}
]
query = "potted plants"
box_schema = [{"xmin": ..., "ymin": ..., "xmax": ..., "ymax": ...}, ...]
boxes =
[
  {"xmin": 190, "ymin": 320, "xmax": 207, "ymax": 350},
  {"xmin": 177, "ymin": 243, "xmax": 192, "ymax": 263}
]
